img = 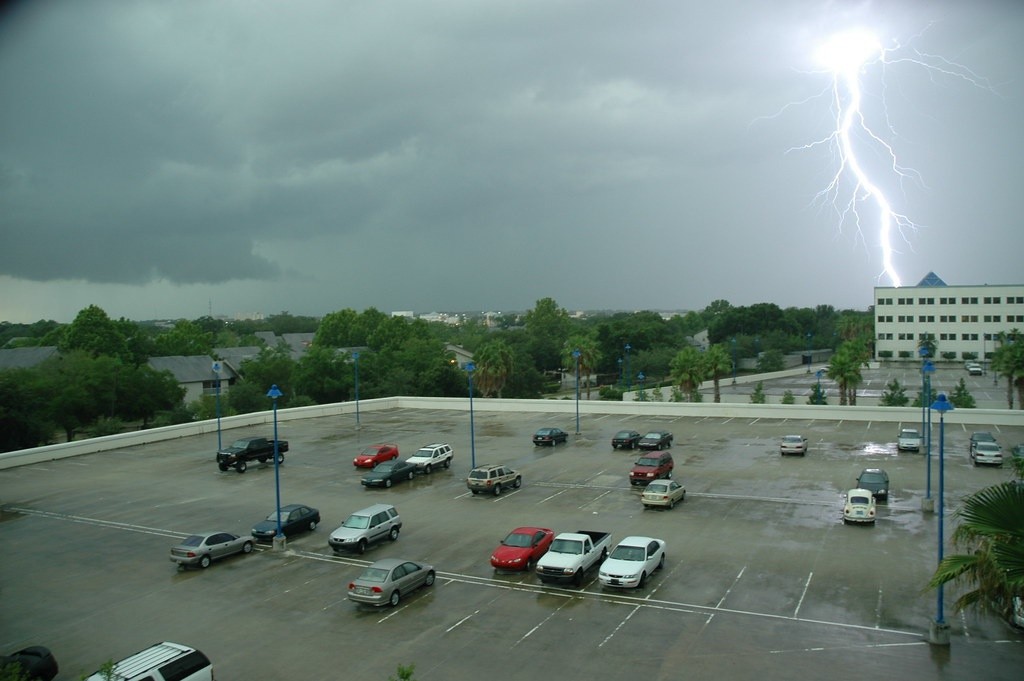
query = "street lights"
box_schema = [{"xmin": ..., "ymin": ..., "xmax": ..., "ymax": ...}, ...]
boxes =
[
  {"xmin": 618, "ymin": 343, "xmax": 646, "ymax": 401},
  {"xmin": 918, "ymin": 345, "xmax": 937, "ymax": 513},
  {"xmin": 266, "ymin": 383, "xmax": 285, "ymax": 552},
  {"xmin": 210, "ymin": 362, "xmax": 221, "ymax": 450},
  {"xmin": 352, "ymin": 351, "xmax": 363, "ymax": 432},
  {"xmin": 570, "ymin": 347, "xmax": 583, "ymax": 440},
  {"xmin": 462, "ymin": 361, "xmax": 476, "ymax": 472},
  {"xmin": 930, "ymin": 393, "xmax": 954, "ymax": 645},
  {"xmin": 981, "ymin": 332, "xmax": 1000, "ymax": 386},
  {"xmin": 730, "ymin": 330, "xmax": 839, "ymax": 405}
]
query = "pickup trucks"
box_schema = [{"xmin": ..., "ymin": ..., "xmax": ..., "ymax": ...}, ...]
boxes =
[
  {"xmin": 638, "ymin": 431, "xmax": 673, "ymax": 451},
  {"xmin": 215, "ymin": 436, "xmax": 290, "ymax": 474},
  {"xmin": 534, "ymin": 529, "xmax": 613, "ymax": 588}
]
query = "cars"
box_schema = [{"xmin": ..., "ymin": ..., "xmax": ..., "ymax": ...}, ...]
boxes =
[
  {"xmin": 819, "ymin": 365, "xmax": 832, "ymax": 373},
  {"xmin": 963, "ymin": 359, "xmax": 983, "ymax": 376},
  {"xmin": 353, "ymin": 444, "xmax": 399, "ymax": 471},
  {"xmin": 968, "ymin": 431, "xmax": 1004, "ymax": 467},
  {"xmin": 897, "ymin": 429, "xmax": 923, "ymax": 453},
  {"xmin": 490, "ymin": 525, "xmax": 555, "ymax": 572},
  {"xmin": 597, "ymin": 536, "xmax": 667, "ymax": 588},
  {"xmin": 842, "ymin": 487, "xmax": 876, "ymax": 524},
  {"xmin": 641, "ymin": 479, "xmax": 687, "ymax": 510},
  {"xmin": 780, "ymin": 434, "xmax": 808, "ymax": 457},
  {"xmin": 0, "ymin": 646, "xmax": 58, "ymax": 681},
  {"xmin": 359, "ymin": 460, "xmax": 417, "ymax": 489},
  {"xmin": 856, "ymin": 468, "xmax": 890, "ymax": 500},
  {"xmin": 168, "ymin": 531, "xmax": 256, "ymax": 568},
  {"xmin": 345, "ymin": 556, "xmax": 436, "ymax": 607},
  {"xmin": 252, "ymin": 503, "xmax": 321, "ymax": 544},
  {"xmin": 532, "ymin": 427, "xmax": 569, "ymax": 446},
  {"xmin": 610, "ymin": 429, "xmax": 642, "ymax": 449}
]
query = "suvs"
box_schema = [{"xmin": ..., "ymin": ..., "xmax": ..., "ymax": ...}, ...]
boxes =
[
  {"xmin": 327, "ymin": 503, "xmax": 402, "ymax": 555},
  {"xmin": 81, "ymin": 641, "xmax": 215, "ymax": 681},
  {"xmin": 629, "ymin": 450, "xmax": 674, "ymax": 485},
  {"xmin": 404, "ymin": 442, "xmax": 454, "ymax": 475},
  {"xmin": 465, "ymin": 463, "xmax": 522, "ymax": 496}
]
